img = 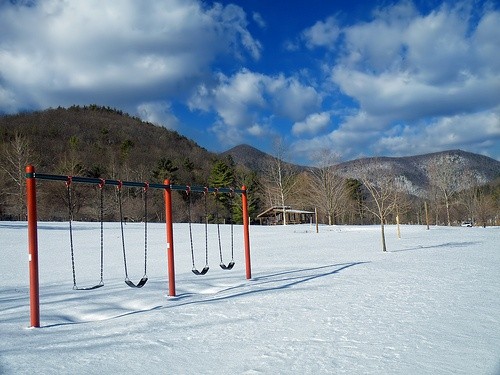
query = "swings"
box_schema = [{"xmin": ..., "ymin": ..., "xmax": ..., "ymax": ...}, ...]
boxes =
[
  {"xmin": 64, "ymin": 175, "xmax": 107, "ymax": 290},
  {"xmin": 115, "ymin": 180, "xmax": 148, "ymax": 288},
  {"xmin": 185, "ymin": 186, "xmax": 211, "ymax": 275},
  {"xmin": 214, "ymin": 186, "xmax": 236, "ymax": 270}
]
